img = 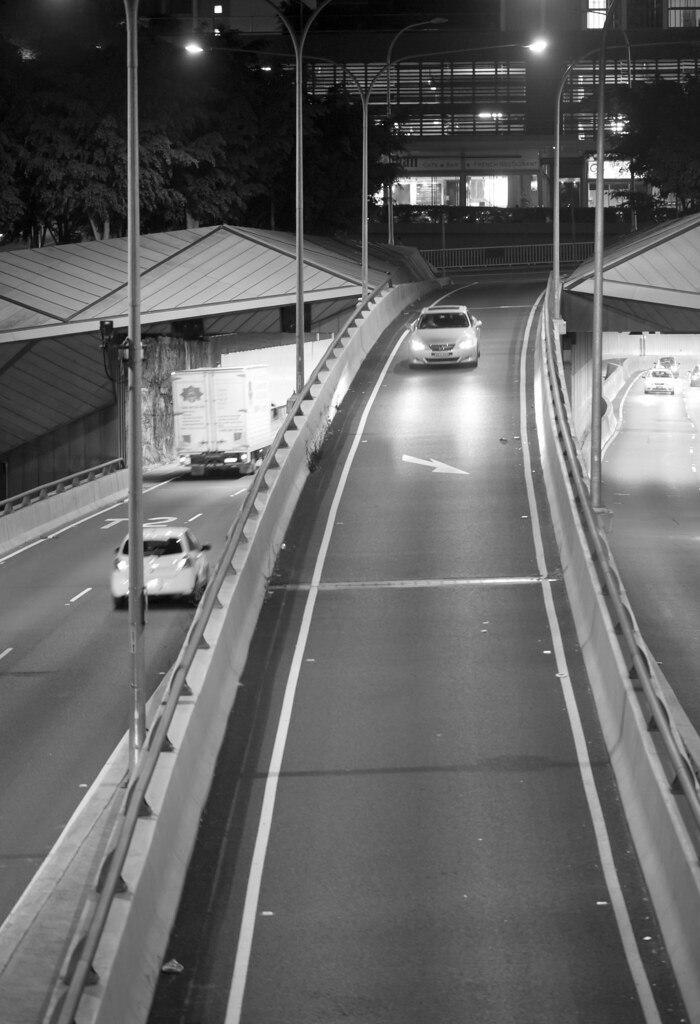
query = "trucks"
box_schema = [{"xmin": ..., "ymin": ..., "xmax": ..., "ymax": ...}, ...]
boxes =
[{"xmin": 170, "ymin": 364, "xmax": 289, "ymax": 477}]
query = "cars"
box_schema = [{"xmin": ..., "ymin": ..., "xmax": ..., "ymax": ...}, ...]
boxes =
[
  {"xmin": 641, "ymin": 367, "xmax": 675, "ymax": 395},
  {"xmin": 688, "ymin": 363, "xmax": 700, "ymax": 387},
  {"xmin": 653, "ymin": 355, "xmax": 681, "ymax": 378},
  {"xmin": 409, "ymin": 305, "xmax": 481, "ymax": 367},
  {"xmin": 111, "ymin": 527, "xmax": 211, "ymax": 609}
]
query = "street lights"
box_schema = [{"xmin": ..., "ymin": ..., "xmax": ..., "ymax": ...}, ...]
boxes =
[
  {"xmin": 386, "ymin": 17, "xmax": 449, "ymax": 244},
  {"xmin": 186, "ymin": 40, "xmax": 548, "ymax": 300}
]
